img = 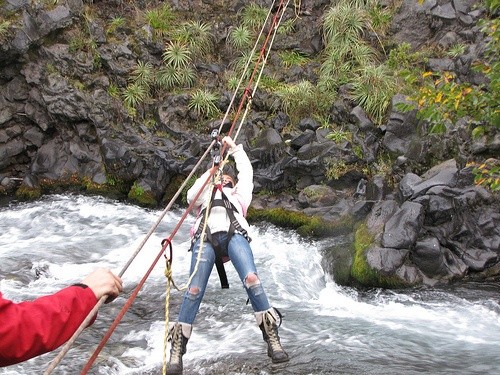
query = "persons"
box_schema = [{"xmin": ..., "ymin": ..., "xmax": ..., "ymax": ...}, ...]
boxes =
[
  {"xmin": 0, "ymin": 267, "xmax": 123, "ymax": 368},
  {"xmin": 166, "ymin": 136, "xmax": 290, "ymax": 375}
]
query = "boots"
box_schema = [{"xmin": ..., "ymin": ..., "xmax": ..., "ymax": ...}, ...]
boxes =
[
  {"xmin": 253, "ymin": 308, "xmax": 290, "ymax": 364},
  {"xmin": 167, "ymin": 321, "xmax": 192, "ymax": 375}
]
